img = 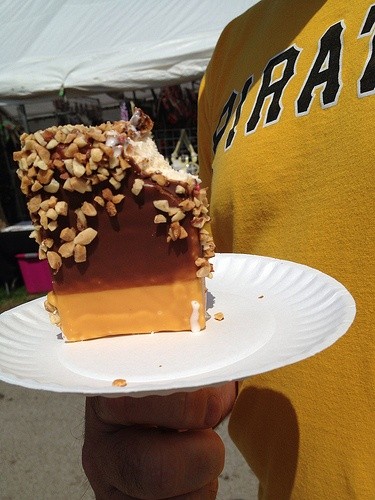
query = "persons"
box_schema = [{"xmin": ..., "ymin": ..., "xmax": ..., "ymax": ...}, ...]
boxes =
[{"xmin": 79, "ymin": 1, "xmax": 375, "ymax": 500}]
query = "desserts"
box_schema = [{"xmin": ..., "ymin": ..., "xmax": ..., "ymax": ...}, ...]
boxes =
[{"xmin": 12, "ymin": 106, "xmax": 223, "ymax": 343}]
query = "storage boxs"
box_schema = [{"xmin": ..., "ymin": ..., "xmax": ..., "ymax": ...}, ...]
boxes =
[{"xmin": 17, "ymin": 253, "xmax": 54, "ymax": 294}]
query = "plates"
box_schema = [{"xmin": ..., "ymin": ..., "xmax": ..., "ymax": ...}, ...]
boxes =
[{"xmin": 0, "ymin": 254, "xmax": 357, "ymax": 397}]
